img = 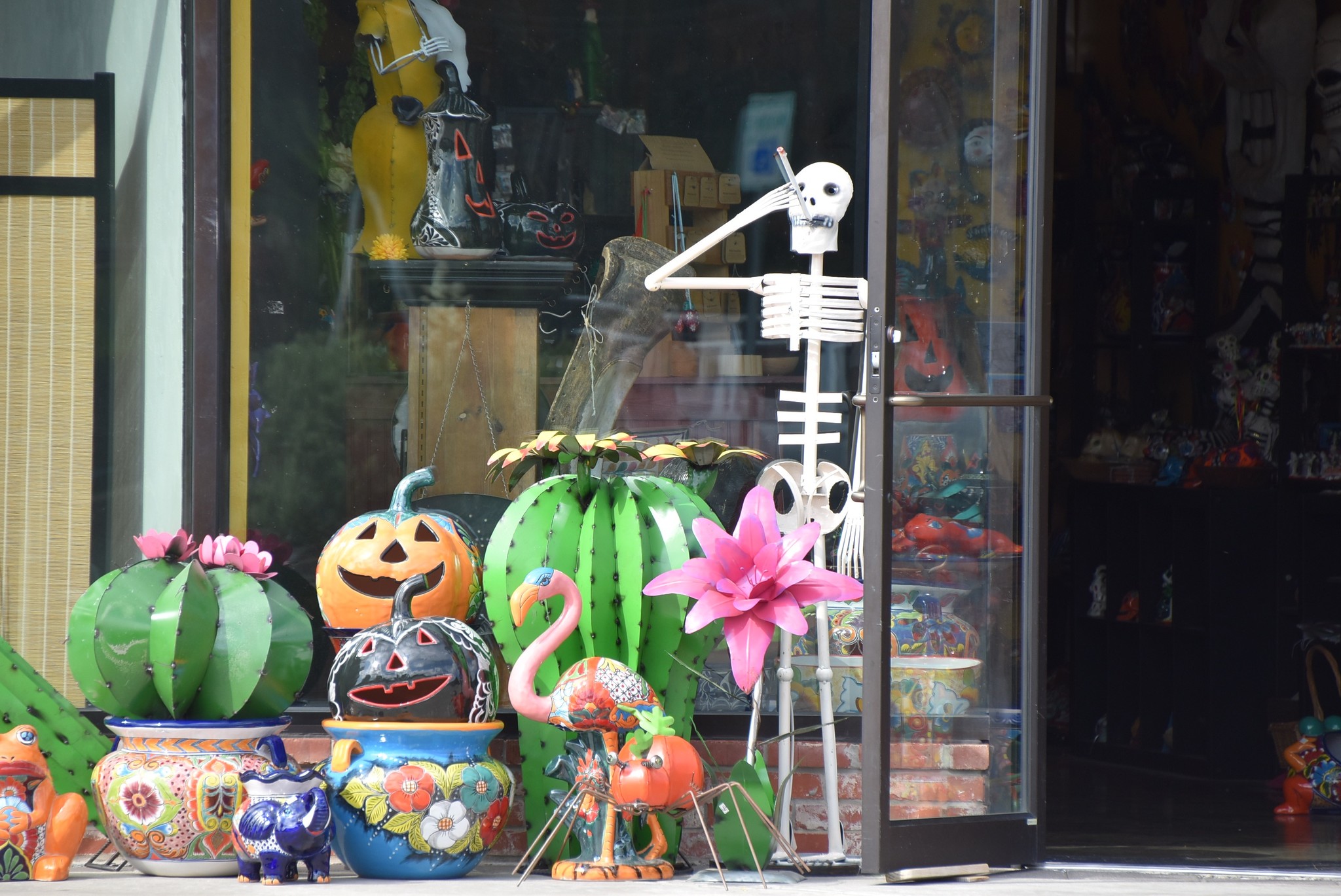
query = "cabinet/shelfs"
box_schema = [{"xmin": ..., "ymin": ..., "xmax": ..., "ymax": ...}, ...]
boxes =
[{"xmin": 1046, "ymin": 475, "xmax": 1340, "ymax": 794}]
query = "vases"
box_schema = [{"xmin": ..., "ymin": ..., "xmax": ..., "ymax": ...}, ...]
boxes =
[
  {"xmin": 89, "ymin": 714, "xmax": 304, "ymax": 877},
  {"xmin": 230, "ymin": 766, "xmax": 337, "ymax": 884},
  {"xmin": 313, "ymin": 718, "xmax": 518, "ymax": 880}
]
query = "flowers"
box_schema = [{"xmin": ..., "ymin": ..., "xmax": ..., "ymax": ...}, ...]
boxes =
[
  {"xmin": 639, "ymin": 482, "xmax": 868, "ymax": 873},
  {"xmin": 65, "ymin": 524, "xmax": 317, "ymax": 726}
]
